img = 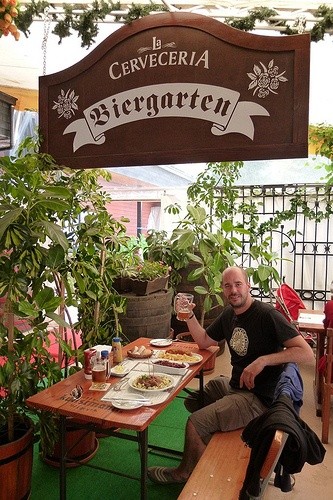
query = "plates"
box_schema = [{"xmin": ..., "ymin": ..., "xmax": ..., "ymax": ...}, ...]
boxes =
[
  {"xmin": 111, "ymin": 394, "xmax": 145, "ymax": 410},
  {"xmin": 155, "ymin": 351, "xmax": 203, "ymax": 364},
  {"xmin": 150, "ymin": 358, "xmax": 190, "ymax": 369},
  {"xmin": 129, "ymin": 374, "xmax": 175, "ymax": 392},
  {"xmin": 150, "ymin": 338, "xmax": 172, "ymax": 347}
]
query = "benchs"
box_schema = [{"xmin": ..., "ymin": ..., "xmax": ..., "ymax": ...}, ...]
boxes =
[{"xmin": 176, "ymin": 362, "xmax": 295, "ymax": 500}]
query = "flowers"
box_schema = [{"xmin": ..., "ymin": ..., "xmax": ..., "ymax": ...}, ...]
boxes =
[{"xmin": 132, "ymin": 260, "xmax": 168, "ymax": 280}]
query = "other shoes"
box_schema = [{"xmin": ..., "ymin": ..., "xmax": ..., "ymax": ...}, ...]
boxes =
[{"xmin": 148, "ymin": 467, "xmax": 189, "ymax": 485}]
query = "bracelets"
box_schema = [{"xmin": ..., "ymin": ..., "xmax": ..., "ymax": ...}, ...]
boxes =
[{"xmin": 190, "ymin": 313, "xmax": 195, "ymax": 319}]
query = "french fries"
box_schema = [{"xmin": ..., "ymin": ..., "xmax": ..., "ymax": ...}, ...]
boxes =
[{"xmin": 159, "ymin": 352, "xmax": 199, "ymax": 362}]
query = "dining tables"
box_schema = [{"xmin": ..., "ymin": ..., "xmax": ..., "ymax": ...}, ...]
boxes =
[{"xmin": 26, "ymin": 337, "xmax": 220, "ymax": 500}]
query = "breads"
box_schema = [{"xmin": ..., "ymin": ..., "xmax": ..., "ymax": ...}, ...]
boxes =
[
  {"xmin": 165, "ymin": 348, "xmax": 192, "ymax": 356},
  {"xmin": 131, "ymin": 345, "xmax": 151, "ymax": 355}
]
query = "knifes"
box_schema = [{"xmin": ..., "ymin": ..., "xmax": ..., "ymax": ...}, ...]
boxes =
[{"xmin": 103, "ymin": 397, "xmax": 150, "ymax": 402}]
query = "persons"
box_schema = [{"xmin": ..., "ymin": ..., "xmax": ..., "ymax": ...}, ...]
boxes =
[{"xmin": 145, "ymin": 266, "xmax": 314, "ymax": 486}]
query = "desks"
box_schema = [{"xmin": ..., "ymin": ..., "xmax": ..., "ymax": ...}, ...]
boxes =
[{"xmin": 298, "ymin": 309, "xmax": 333, "ymax": 417}]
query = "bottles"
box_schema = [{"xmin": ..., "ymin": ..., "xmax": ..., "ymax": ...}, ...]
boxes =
[
  {"xmin": 100, "ymin": 350, "xmax": 110, "ymax": 380},
  {"xmin": 112, "ymin": 337, "xmax": 123, "ymax": 363}
]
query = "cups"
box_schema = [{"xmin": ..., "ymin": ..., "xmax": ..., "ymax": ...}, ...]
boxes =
[
  {"xmin": 173, "ymin": 293, "xmax": 195, "ymax": 321},
  {"xmin": 90, "ymin": 356, "xmax": 111, "ymax": 389}
]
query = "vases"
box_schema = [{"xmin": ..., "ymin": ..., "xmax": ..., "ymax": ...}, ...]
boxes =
[{"xmin": 125, "ymin": 275, "xmax": 171, "ymax": 296}]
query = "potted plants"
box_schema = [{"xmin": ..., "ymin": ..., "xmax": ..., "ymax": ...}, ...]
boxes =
[{"xmin": 0, "ymin": 136, "xmax": 295, "ymax": 500}]
